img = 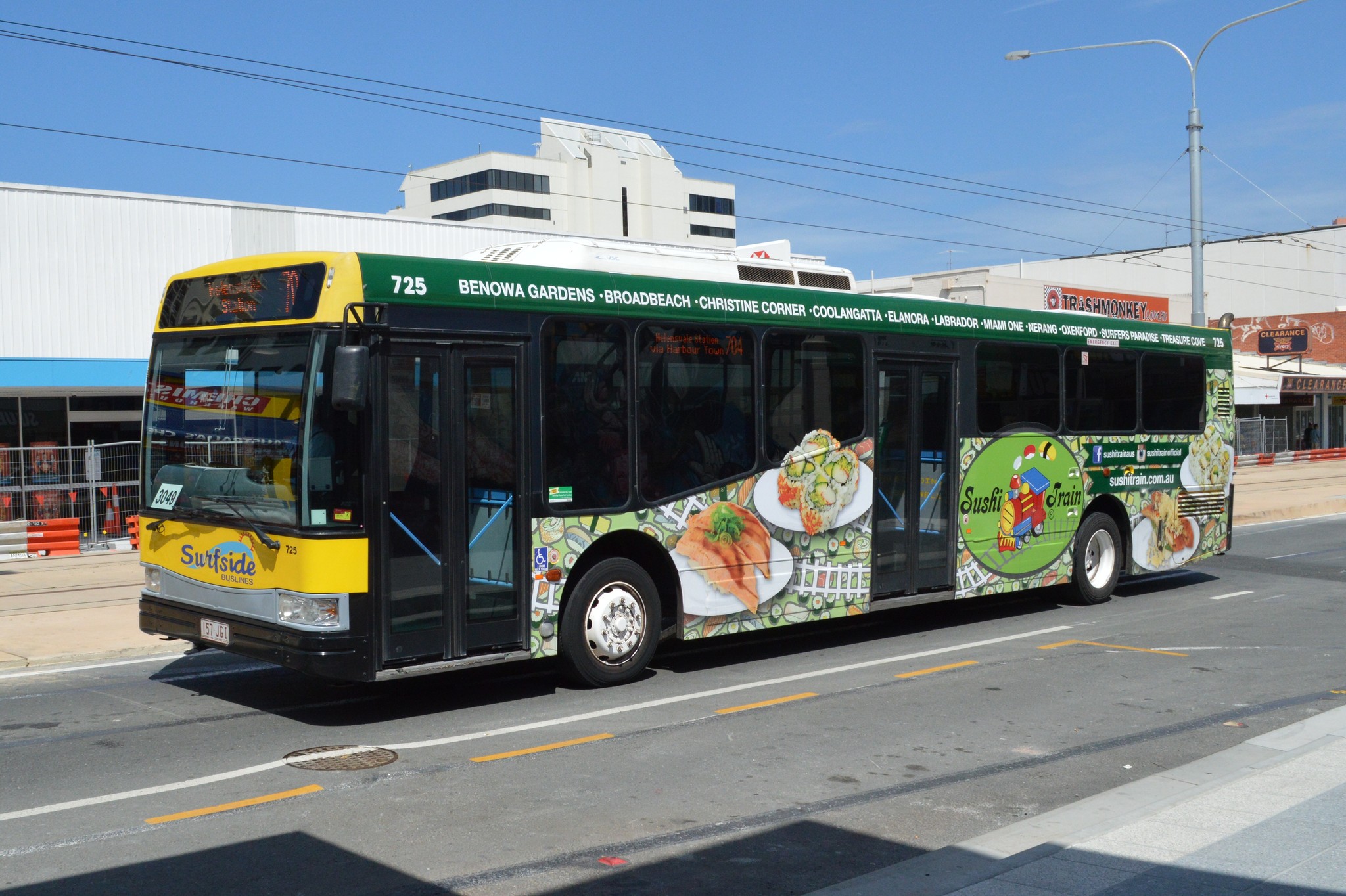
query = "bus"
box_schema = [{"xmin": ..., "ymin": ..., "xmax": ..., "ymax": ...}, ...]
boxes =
[{"xmin": 139, "ymin": 238, "xmax": 1235, "ymax": 685}]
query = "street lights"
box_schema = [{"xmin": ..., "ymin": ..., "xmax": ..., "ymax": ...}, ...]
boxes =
[{"xmin": 1005, "ymin": 0, "xmax": 1308, "ymax": 326}]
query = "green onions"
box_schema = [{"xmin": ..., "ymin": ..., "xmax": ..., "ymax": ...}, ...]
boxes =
[{"xmin": 702, "ymin": 503, "xmax": 747, "ymax": 546}]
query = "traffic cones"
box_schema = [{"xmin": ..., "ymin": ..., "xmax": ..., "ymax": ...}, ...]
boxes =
[{"xmin": 98, "ymin": 500, "xmax": 115, "ymax": 534}]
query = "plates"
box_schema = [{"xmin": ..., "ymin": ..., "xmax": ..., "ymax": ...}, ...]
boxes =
[
  {"xmin": 667, "ymin": 537, "xmax": 794, "ymax": 615},
  {"xmin": 753, "ymin": 458, "xmax": 873, "ymax": 532},
  {"xmin": 1132, "ymin": 512, "xmax": 1201, "ymax": 570},
  {"xmin": 1180, "ymin": 443, "xmax": 1234, "ymax": 500}
]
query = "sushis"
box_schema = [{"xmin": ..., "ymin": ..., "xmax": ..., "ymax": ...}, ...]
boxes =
[
  {"xmin": 777, "ymin": 429, "xmax": 871, "ymax": 563},
  {"xmin": 1188, "ymin": 425, "xmax": 1234, "ymax": 486}
]
